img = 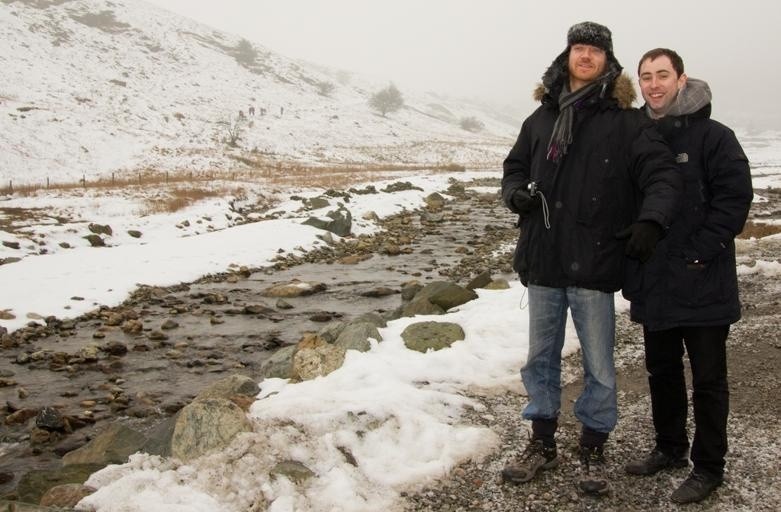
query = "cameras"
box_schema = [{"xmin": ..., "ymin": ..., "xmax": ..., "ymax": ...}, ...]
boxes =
[{"xmin": 526, "ymin": 182, "xmax": 537, "ymax": 197}]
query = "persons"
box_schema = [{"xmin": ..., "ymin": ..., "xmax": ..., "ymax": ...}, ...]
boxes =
[
  {"xmin": 499, "ymin": 18, "xmax": 680, "ymax": 498},
  {"xmin": 621, "ymin": 46, "xmax": 754, "ymax": 507}
]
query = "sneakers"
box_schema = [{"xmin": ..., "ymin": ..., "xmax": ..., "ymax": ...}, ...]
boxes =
[
  {"xmin": 669, "ymin": 462, "xmax": 726, "ymax": 503},
  {"xmin": 622, "ymin": 449, "xmax": 690, "ymax": 477},
  {"xmin": 499, "ymin": 434, "xmax": 559, "ymax": 484},
  {"xmin": 574, "ymin": 443, "xmax": 612, "ymax": 496}
]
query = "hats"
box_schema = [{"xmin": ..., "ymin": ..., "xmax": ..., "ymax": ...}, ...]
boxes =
[{"xmin": 565, "ymin": 19, "xmax": 614, "ymax": 55}]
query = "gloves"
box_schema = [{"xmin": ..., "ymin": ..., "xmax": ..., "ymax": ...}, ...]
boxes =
[
  {"xmin": 613, "ymin": 218, "xmax": 663, "ymax": 264},
  {"xmin": 510, "ymin": 187, "xmax": 541, "ymax": 214}
]
